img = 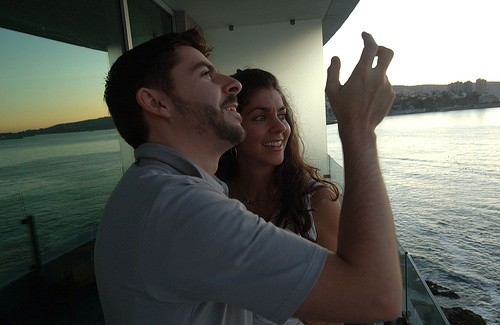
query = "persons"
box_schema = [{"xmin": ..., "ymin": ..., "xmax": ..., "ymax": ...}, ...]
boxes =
[
  {"xmin": 94, "ymin": 28, "xmax": 404, "ymax": 325},
  {"xmin": 213, "ymin": 68, "xmax": 341, "ymax": 325}
]
